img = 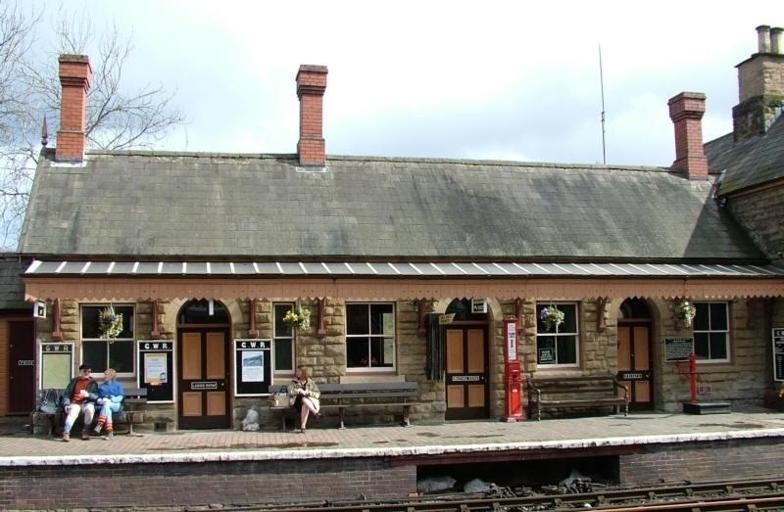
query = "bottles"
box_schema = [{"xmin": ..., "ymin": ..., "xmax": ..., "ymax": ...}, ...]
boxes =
[{"xmin": 79, "ymin": 364, "xmax": 92, "ymax": 370}]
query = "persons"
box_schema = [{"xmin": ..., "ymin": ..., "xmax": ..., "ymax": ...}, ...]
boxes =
[
  {"xmin": 59, "ymin": 364, "xmax": 100, "ymax": 442},
  {"xmin": 287, "ymin": 364, "xmax": 322, "ymax": 433},
  {"xmin": 94, "ymin": 368, "xmax": 125, "ymax": 440}
]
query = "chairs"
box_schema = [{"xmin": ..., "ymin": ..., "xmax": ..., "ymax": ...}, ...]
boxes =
[
  {"xmin": 62, "ymin": 431, "xmax": 71, "ymax": 442},
  {"xmin": 94, "ymin": 423, "xmax": 104, "ymax": 434},
  {"xmin": 314, "ymin": 413, "xmax": 325, "ymax": 420},
  {"xmin": 301, "ymin": 424, "xmax": 307, "ymax": 433},
  {"xmin": 104, "ymin": 430, "xmax": 115, "ymax": 440},
  {"xmin": 81, "ymin": 431, "xmax": 90, "ymax": 440}
]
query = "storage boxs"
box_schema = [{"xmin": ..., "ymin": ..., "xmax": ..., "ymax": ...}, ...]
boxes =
[
  {"xmin": 527, "ymin": 375, "xmax": 628, "ymax": 421},
  {"xmin": 270, "ymin": 382, "xmax": 421, "ymax": 432},
  {"xmin": 39, "ymin": 389, "xmax": 148, "ymax": 437}
]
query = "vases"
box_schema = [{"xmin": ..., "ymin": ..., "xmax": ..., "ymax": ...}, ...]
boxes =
[
  {"xmin": 541, "ymin": 306, "xmax": 564, "ymax": 331},
  {"xmin": 674, "ymin": 299, "xmax": 696, "ymax": 327},
  {"xmin": 98, "ymin": 304, "xmax": 123, "ymax": 339},
  {"xmin": 283, "ymin": 309, "xmax": 311, "ymax": 333}
]
